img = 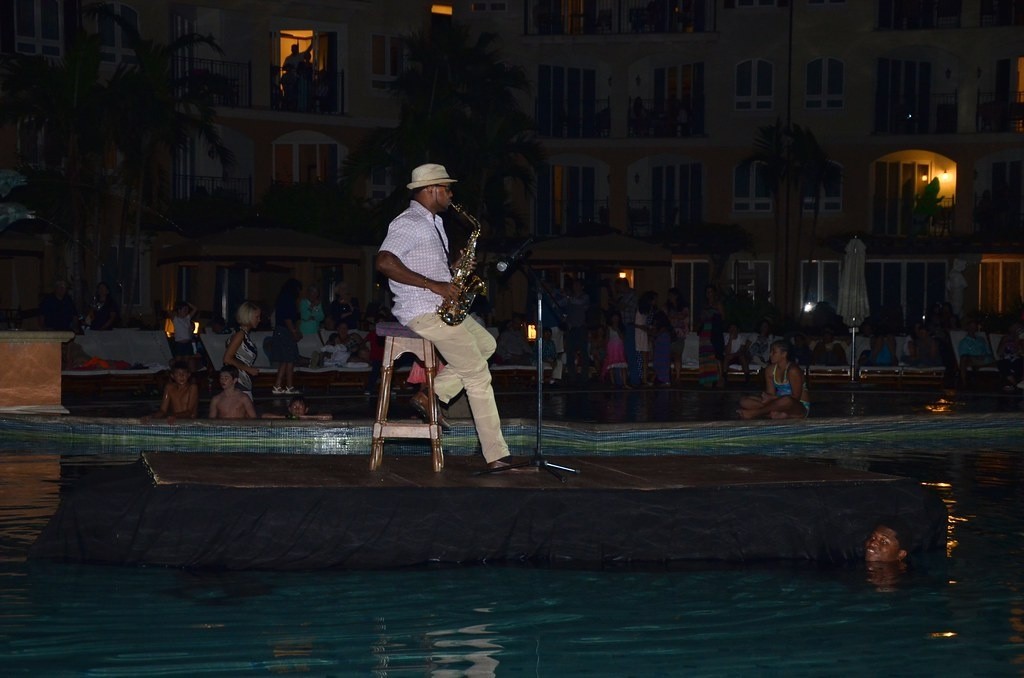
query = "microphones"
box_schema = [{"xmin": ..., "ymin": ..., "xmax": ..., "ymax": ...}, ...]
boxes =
[{"xmin": 497, "ymin": 236, "xmax": 535, "ymax": 272}]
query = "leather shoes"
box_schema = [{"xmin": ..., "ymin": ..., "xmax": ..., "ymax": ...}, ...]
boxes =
[
  {"xmin": 487, "ymin": 455, "xmax": 532, "ymax": 472},
  {"xmin": 409, "ymin": 390, "xmax": 450, "ymax": 430}
]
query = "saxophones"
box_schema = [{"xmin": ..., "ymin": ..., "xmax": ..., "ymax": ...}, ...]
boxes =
[{"xmin": 436, "ymin": 201, "xmax": 488, "ymax": 327}]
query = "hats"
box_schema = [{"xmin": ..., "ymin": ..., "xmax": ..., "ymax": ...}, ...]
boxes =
[{"xmin": 406, "ymin": 164, "xmax": 458, "ymax": 190}]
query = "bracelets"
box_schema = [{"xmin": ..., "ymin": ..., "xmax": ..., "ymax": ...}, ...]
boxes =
[{"xmin": 424, "ymin": 276, "xmax": 427, "ymax": 291}]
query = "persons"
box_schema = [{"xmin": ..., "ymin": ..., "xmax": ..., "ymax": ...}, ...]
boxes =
[
  {"xmin": 374, "ymin": 163, "xmax": 539, "ymax": 476},
  {"xmin": 865, "ymin": 517, "xmax": 910, "ymax": 562},
  {"xmin": 35, "ymin": 278, "xmax": 118, "ymax": 335},
  {"xmin": 271, "ymin": 36, "xmax": 318, "ymax": 111},
  {"xmin": 864, "ymin": 563, "xmax": 907, "ymax": 593},
  {"xmin": 141, "ymin": 271, "xmax": 1006, "ymax": 420}
]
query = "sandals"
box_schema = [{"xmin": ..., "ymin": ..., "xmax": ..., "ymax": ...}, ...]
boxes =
[{"xmin": 272, "ymin": 386, "xmax": 303, "ymax": 394}]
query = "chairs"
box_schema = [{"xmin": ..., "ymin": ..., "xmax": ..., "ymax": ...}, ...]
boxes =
[
  {"xmin": 947, "ymin": 330, "xmax": 999, "ymax": 393},
  {"xmin": 723, "ymin": 332, "xmax": 762, "ymax": 389},
  {"xmin": 293, "ymin": 329, "xmax": 336, "ymax": 392},
  {"xmin": 486, "ymin": 326, "xmax": 538, "ymax": 385},
  {"xmin": 127, "ymin": 330, "xmax": 175, "ymax": 396},
  {"xmin": 86, "ymin": 330, "xmax": 154, "ymax": 397},
  {"xmin": 61, "ymin": 335, "xmax": 109, "ymax": 399},
  {"xmin": 808, "ymin": 338, "xmax": 853, "ymax": 389},
  {"xmin": 249, "ymin": 331, "xmax": 279, "ymax": 393},
  {"xmin": 988, "ymin": 334, "xmax": 1024, "ymax": 389},
  {"xmin": 855, "ymin": 335, "xmax": 902, "ymax": 391},
  {"xmin": 670, "ymin": 331, "xmax": 700, "ymax": 381},
  {"xmin": 771, "ymin": 336, "xmax": 807, "ymax": 389},
  {"xmin": 895, "ymin": 336, "xmax": 945, "ymax": 392},
  {"xmin": 199, "ymin": 332, "xmax": 232, "ymax": 391},
  {"xmin": 321, "ymin": 328, "xmax": 372, "ymax": 392}
]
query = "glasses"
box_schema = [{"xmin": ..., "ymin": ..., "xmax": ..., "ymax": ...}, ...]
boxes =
[{"xmin": 435, "ymin": 185, "xmax": 450, "ymax": 192}]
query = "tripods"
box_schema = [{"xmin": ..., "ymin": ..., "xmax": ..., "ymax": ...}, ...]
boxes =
[{"xmin": 471, "ymin": 261, "xmax": 580, "ymax": 485}]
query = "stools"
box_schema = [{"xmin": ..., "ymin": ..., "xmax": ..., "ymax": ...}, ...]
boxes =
[{"xmin": 367, "ymin": 321, "xmax": 444, "ymax": 472}]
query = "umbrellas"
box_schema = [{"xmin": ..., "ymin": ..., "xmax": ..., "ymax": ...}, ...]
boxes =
[{"xmin": 837, "ymin": 237, "xmax": 870, "ymax": 385}]
